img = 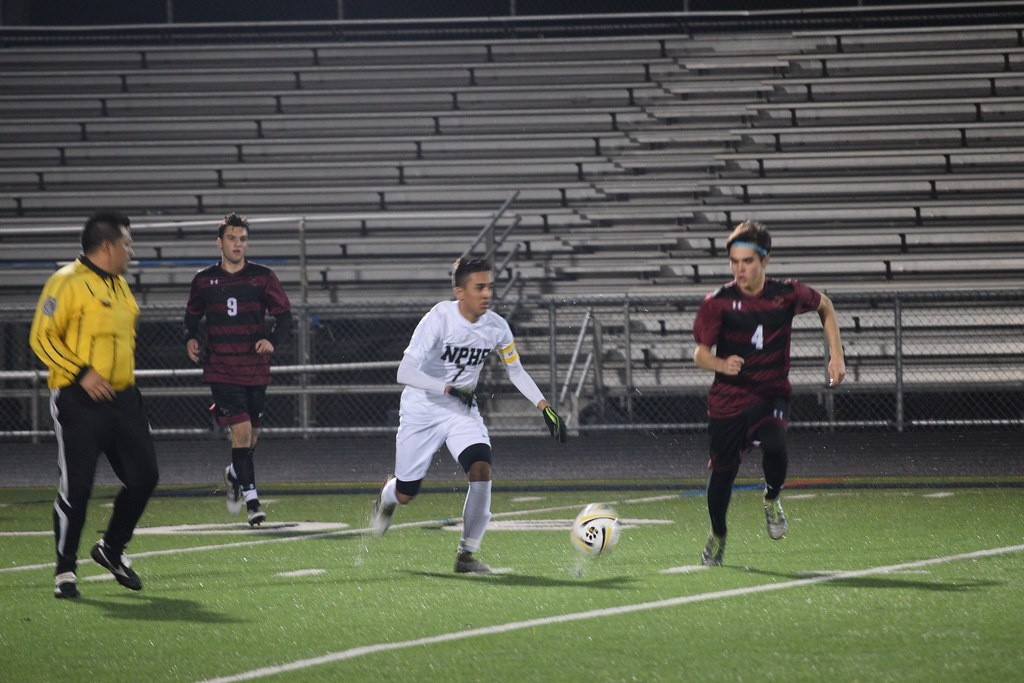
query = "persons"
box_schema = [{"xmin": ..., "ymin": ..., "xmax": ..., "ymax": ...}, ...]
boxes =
[
  {"xmin": 181, "ymin": 214, "xmax": 292, "ymax": 528},
  {"xmin": 30, "ymin": 209, "xmax": 160, "ymax": 598},
  {"xmin": 692, "ymin": 219, "xmax": 845, "ymax": 567},
  {"xmin": 374, "ymin": 257, "xmax": 567, "ymax": 573}
]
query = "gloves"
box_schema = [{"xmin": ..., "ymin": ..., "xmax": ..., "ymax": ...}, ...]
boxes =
[
  {"xmin": 448, "ymin": 385, "xmax": 478, "ymax": 408},
  {"xmin": 542, "ymin": 406, "xmax": 567, "ymax": 444}
]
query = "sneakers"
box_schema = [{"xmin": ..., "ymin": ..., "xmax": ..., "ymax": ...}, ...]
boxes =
[
  {"xmin": 454, "ymin": 550, "xmax": 492, "ymax": 573},
  {"xmin": 371, "ymin": 474, "xmax": 397, "ymax": 537},
  {"xmin": 702, "ymin": 528, "xmax": 728, "ymax": 566},
  {"xmin": 762, "ymin": 488, "xmax": 787, "ymax": 540},
  {"xmin": 247, "ymin": 502, "xmax": 267, "ymax": 528},
  {"xmin": 224, "ymin": 466, "xmax": 242, "ymax": 515},
  {"xmin": 54, "ymin": 571, "xmax": 81, "ymax": 598},
  {"xmin": 90, "ymin": 538, "xmax": 143, "ymax": 591}
]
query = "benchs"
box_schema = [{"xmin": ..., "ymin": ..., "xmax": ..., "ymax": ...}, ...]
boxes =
[{"xmin": 0, "ymin": 0, "xmax": 1024, "ymax": 395}]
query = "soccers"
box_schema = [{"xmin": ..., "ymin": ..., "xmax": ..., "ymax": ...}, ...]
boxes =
[{"xmin": 571, "ymin": 503, "xmax": 622, "ymax": 556}]
